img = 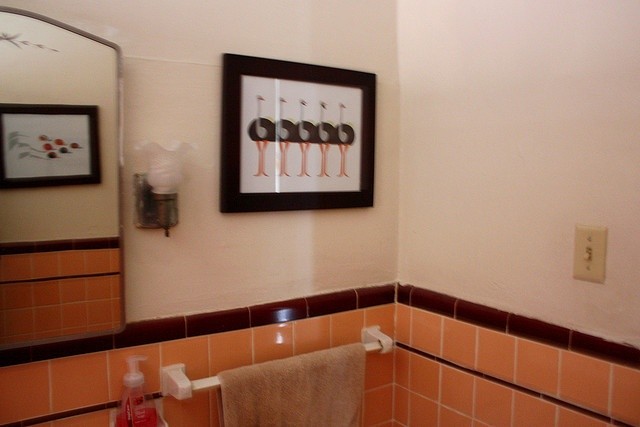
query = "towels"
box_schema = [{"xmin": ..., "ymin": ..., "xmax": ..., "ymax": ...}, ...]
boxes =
[{"xmin": 211, "ymin": 340, "xmax": 368, "ymax": 426}]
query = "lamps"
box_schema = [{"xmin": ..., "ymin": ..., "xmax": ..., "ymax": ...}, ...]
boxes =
[{"xmin": 131, "ymin": 139, "xmax": 200, "ymax": 232}]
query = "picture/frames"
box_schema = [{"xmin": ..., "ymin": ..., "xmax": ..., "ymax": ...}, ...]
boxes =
[
  {"xmin": 220, "ymin": 51, "xmax": 378, "ymax": 213},
  {"xmin": 0, "ymin": 101, "xmax": 102, "ymax": 189}
]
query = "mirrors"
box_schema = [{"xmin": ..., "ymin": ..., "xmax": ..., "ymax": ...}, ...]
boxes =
[{"xmin": 0, "ymin": 4, "xmax": 127, "ymax": 349}]
